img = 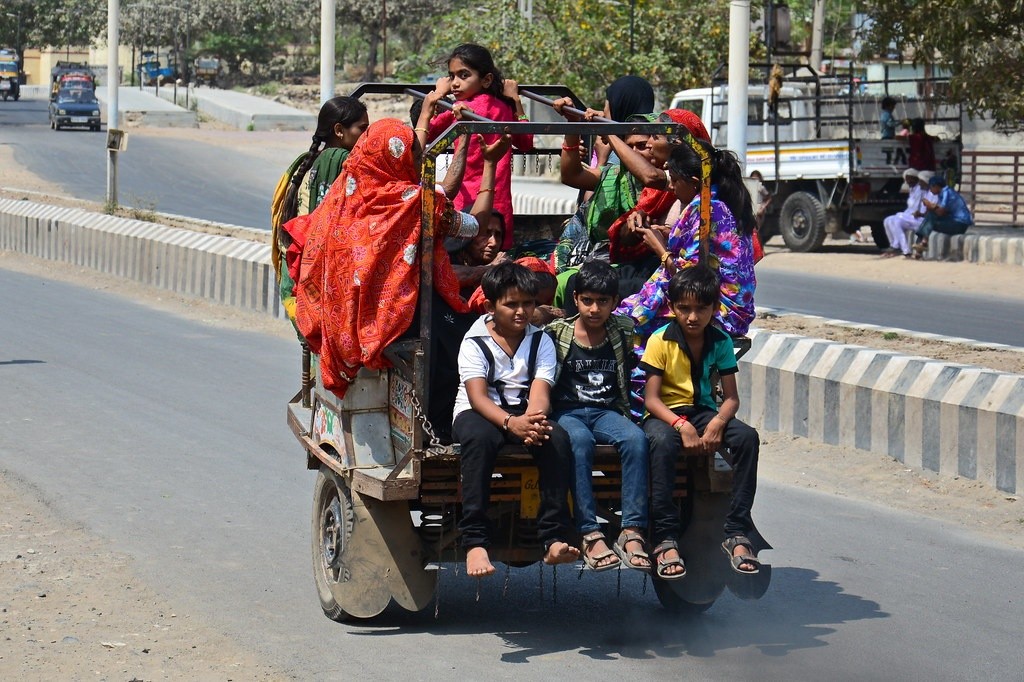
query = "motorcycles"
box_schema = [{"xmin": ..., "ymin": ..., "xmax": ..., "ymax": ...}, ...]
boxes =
[
  {"xmin": 50, "ymin": 61, "xmax": 97, "ymax": 94},
  {"xmin": 0, "ymin": 48, "xmax": 19, "ymax": 102},
  {"xmin": 271, "ymin": 84, "xmax": 739, "ymax": 625},
  {"xmin": 136, "ymin": 50, "xmax": 222, "ymax": 87}
]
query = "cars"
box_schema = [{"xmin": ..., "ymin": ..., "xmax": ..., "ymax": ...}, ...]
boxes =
[{"xmin": 48, "ymin": 88, "xmax": 102, "ymax": 131}]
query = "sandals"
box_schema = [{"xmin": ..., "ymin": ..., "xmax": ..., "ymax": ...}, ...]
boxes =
[
  {"xmin": 613, "ymin": 531, "xmax": 652, "ymax": 570},
  {"xmin": 652, "ymin": 539, "xmax": 687, "ymax": 580},
  {"xmin": 581, "ymin": 531, "xmax": 621, "ymax": 572},
  {"xmin": 721, "ymin": 535, "xmax": 759, "ymax": 574}
]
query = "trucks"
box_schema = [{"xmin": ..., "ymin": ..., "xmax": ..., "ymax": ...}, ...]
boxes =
[{"xmin": 666, "ymin": 85, "xmax": 966, "ymax": 251}]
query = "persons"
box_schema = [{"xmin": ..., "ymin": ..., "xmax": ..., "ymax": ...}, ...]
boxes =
[
  {"xmin": 282, "ymin": 102, "xmax": 512, "ymax": 401},
  {"xmin": 427, "ymin": 44, "xmax": 763, "ymax": 326},
  {"xmin": 880, "ymin": 96, "xmax": 900, "ymax": 140},
  {"xmin": 638, "ymin": 265, "xmax": 760, "ymax": 581},
  {"xmin": 272, "ymin": 90, "xmax": 444, "ymax": 346},
  {"xmin": 541, "ymin": 260, "xmax": 653, "ymax": 572},
  {"xmin": 896, "ymin": 117, "xmax": 941, "ymax": 170},
  {"xmin": 883, "ymin": 168, "xmax": 971, "ymax": 259},
  {"xmin": 454, "ymin": 261, "xmax": 580, "ymax": 575},
  {"xmin": 615, "ymin": 142, "xmax": 759, "ymax": 420}
]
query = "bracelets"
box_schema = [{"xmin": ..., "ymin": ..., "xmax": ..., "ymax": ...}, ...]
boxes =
[
  {"xmin": 671, "ymin": 416, "xmax": 687, "ymax": 431},
  {"xmin": 477, "ymin": 188, "xmax": 494, "ymax": 195},
  {"xmin": 502, "ymin": 414, "xmax": 515, "ymax": 431},
  {"xmin": 415, "ymin": 128, "xmax": 430, "ymax": 137},
  {"xmin": 562, "ymin": 143, "xmax": 580, "ymax": 150},
  {"xmin": 661, "ymin": 251, "xmax": 672, "ymax": 267}
]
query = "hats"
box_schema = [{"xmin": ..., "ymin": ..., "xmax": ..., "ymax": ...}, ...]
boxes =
[
  {"xmin": 908, "ymin": 117, "xmax": 926, "ymax": 129},
  {"xmin": 917, "ymin": 170, "xmax": 935, "ymax": 183},
  {"xmin": 902, "ymin": 167, "xmax": 918, "ymax": 180}
]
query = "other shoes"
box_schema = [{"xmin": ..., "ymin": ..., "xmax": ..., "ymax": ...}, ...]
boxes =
[
  {"xmin": 910, "ymin": 254, "xmax": 923, "ymax": 260},
  {"xmin": 913, "ymin": 242, "xmax": 927, "ymax": 251},
  {"xmin": 883, "ymin": 247, "xmax": 902, "ymax": 253}
]
